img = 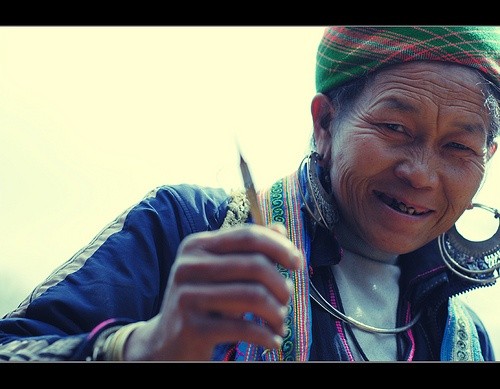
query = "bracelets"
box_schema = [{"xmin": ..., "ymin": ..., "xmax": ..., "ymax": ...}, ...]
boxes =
[{"xmin": 103, "ymin": 318, "xmax": 145, "ymax": 361}]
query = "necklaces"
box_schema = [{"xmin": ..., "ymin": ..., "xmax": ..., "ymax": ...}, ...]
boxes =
[{"xmin": 306, "ymin": 273, "xmax": 430, "ymax": 334}]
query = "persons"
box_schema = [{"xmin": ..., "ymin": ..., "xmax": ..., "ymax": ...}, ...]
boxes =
[{"xmin": 0, "ymin": 24, "xmax": 500, "ymax": 363}]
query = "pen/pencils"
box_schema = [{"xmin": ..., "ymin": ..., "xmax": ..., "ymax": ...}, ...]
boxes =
[{"xmin": 237, "ymin": 147, "xmax": 265, "ymax": 229}]
